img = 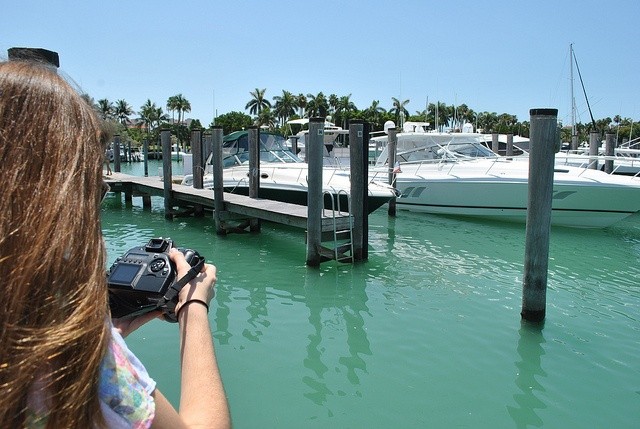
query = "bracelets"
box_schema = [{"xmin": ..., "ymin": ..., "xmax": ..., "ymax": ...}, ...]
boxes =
[{"xmin": 177, "ymin": 299, "xmax": 209, "ymax": 322}]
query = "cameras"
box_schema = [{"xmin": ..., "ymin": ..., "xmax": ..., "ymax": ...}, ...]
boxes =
[{"xmin": 104, "ymin": 236, "xmax": 205, "ymax": 322}]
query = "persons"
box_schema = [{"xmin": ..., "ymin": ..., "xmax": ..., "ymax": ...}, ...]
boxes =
[{"xmin": 1, "ymin": 58, "xmax": 234, "ymax": 428}]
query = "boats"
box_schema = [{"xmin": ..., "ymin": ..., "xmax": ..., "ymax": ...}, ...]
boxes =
[
  {"xmin": 180, "ymin": 127, "xmax": 403, "ymax": 216},
  {"xmin": 335, "ymin": 128, "xmax": 640, "ymax": 231},
  {"xmin": 478, "ymin": 133, "xmax": 640, "ymax": 176}
]
